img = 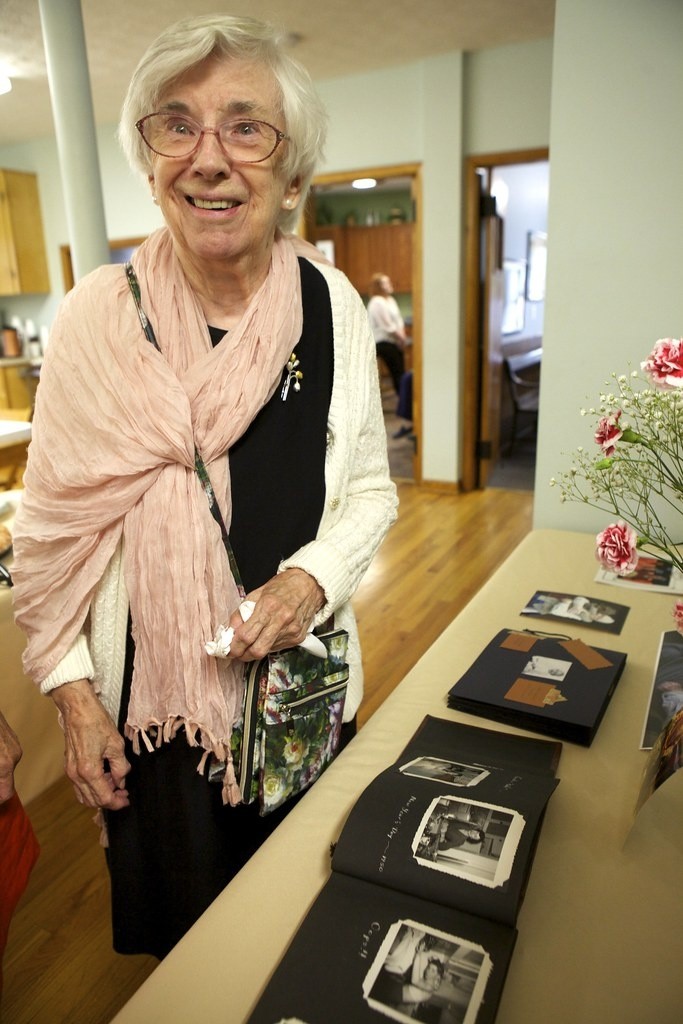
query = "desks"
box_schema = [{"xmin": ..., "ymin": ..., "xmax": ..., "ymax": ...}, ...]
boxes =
[
  {"xmin": 0, "ymin": 420, "xmax": 32, "ymax": 449},
  {"xmin": 113, "ymin": 531, "xmax": 683, "ymax": 1023}
]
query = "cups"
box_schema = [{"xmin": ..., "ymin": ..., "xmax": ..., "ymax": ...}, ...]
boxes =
[{"xmin": 0, "ymin": 328, "xmax": 19, "ymax": 357}]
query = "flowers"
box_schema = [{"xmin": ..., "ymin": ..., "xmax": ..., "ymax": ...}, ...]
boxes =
[{"xmin": 556, "ymin": 337, "xmax": 683, "ymax": 637}]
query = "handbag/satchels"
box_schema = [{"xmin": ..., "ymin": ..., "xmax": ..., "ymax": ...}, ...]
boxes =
[{"xmin": 208, "ymin": 629, "xmax": 350, "ymax": 816}]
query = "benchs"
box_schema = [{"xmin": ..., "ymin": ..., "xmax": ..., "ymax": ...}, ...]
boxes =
[{"xmin": 501, "ymin": 348, "xmax": 542, "ymax": 467}]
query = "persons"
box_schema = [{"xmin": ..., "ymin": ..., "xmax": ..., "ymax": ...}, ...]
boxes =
[
  {"xmin": 368, "ymin": 274, "xmax": 413, "ymax": 439},
  {"xmin": 0, "ymin": 710, "xmax": 41, "ymax": 955},
  {"xmin": 11, "ymin": 12, "xmax": 400, "ymax": 963},
  {"xmin": 525, "ymin": 656, "xmax": 564, "ymax": 676},
  {"xmin": 529, "ymin": 592, "xmax": 615, "ymax": 623},
  {"xmin": 368, "ymin": 942, "xmax": 445, "ymax": 1006},
  {"xmin": 617, "ymin": 557, "xmax": 666, "ymax": 583},
  {"xmin": 439, "ymin": 817, "xmax": 485, "ymax": 850},
  {"xmin": 411, "ymin": 760, "xmax": 464, "ymax": 776}
]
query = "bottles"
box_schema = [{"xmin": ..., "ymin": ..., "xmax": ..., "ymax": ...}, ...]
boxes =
[{"xmin": 28, "ymin": 336, "xmax": 42, "ymax": 358}]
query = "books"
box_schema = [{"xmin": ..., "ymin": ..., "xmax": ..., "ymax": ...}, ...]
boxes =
[
  {"xmin": 246, "ymin": 714, "xmax": 563, "ymax": 1024},
  {"xmin": 447, "ymin": 628, "xmax": 628, "ymax": 749}
]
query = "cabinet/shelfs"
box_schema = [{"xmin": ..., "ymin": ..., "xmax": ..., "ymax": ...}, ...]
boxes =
[
  {"xmin": 313, "ymin": 224, "xmax": 413, "ymax": 294},
  {"xmin": 0, "ymin": 364, "xmax": 39, "ymax": 493},
  {"xmin": 0, "ymin": 166, "xmax": 52, "ymax": 295}
]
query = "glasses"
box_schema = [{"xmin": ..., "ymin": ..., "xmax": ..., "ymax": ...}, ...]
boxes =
[{"xmin": 134, "ymin": 112, "xmax": 292, "ymax": 164}]
